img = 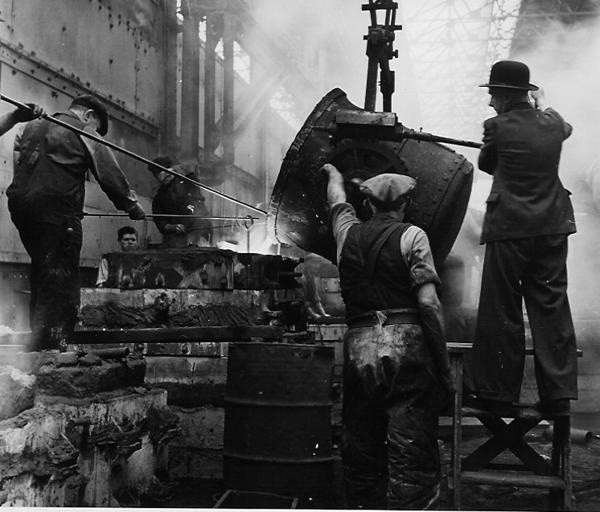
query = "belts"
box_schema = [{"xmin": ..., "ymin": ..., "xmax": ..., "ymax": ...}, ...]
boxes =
[{"xmin": 343, "ymin": 310, "xmax": 421, "ymax": 328}]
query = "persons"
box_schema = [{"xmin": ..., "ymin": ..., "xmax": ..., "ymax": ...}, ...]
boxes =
[
  {"xmin": 96, "ymin": 227, "xmax": 166, "ymax": 290},
  {"xmin": 147, "ymin": 157, "xmax": 214, "ymax": 250},
  {"xmin": 0, "ymin": 103, "xmax": 50, "ymax": 137},
  {"xmin": 6, "ymin": 94, "xmax": 145, "ymax": 351},
  {"xmin": 318, "ymin": 164, "xmax": 457, "ymax": 510},
  {"xmin": 469, "ymin": 60, "xmax": 578, "ymax": 415}
]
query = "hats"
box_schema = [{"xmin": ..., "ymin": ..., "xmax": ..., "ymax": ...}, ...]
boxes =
[
  {"xmin": 359, "ymin": 173, "xmax": 416, "ymax": 209},
  {"xmin": 147, "ymin": 157, "xmax": 172, "ymax": 177},
  {"xmin": 478, "ymin": 60, "xmax": 539, "ymax": 91},
  {"xmin": 71, "ymin": 94, "xmax": 108, "ymax": 137}
]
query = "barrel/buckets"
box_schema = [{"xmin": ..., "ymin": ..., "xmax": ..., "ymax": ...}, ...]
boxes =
[
  {"xmin": 267, "ymin": 87, "xmax": 474, "ymax": 277},
  {"xmin": 223, "ymin": 338, "xmax": 335, "ymax": 506}
]
